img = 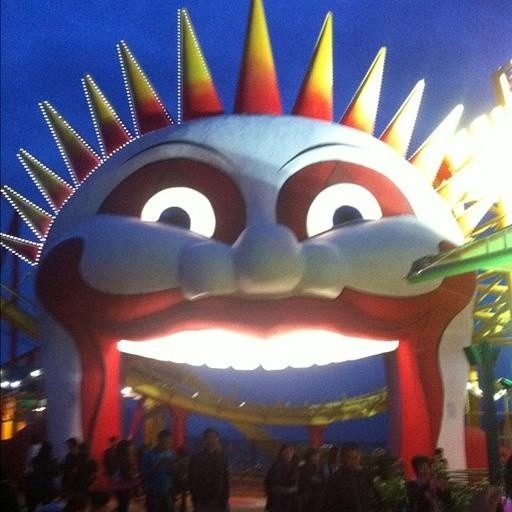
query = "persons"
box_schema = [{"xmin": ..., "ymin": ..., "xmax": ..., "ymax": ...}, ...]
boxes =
[
  {"xmin": 267, "ymin": 440, "xmax": 512, "ymax": 512},
  {"xmin": 0, "ymin": 426, "xmax": 230, "ymax": 512}
]
